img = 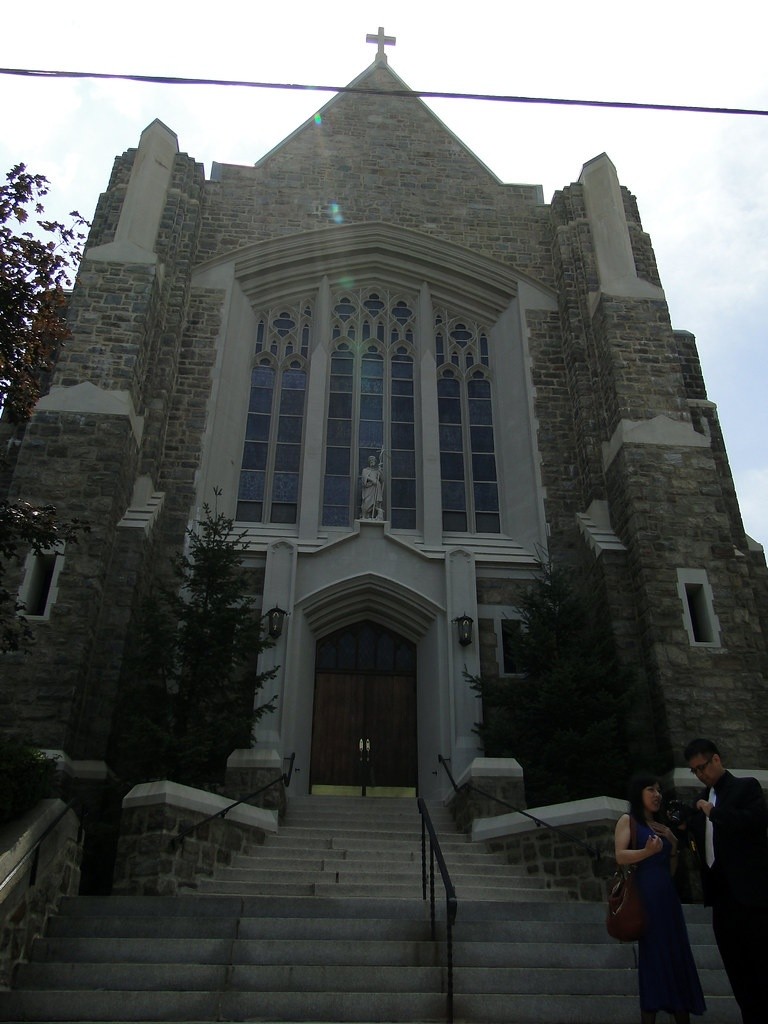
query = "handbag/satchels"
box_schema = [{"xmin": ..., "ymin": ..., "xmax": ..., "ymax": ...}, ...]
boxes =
[{"xmin": 607, "ymin": 868, "xmax": 646, "ymax": 942}]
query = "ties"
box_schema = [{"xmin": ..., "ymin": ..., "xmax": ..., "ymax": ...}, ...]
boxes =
[{"xmin": 705, "ymin": 786, "xmax": 717, "ymax": 868}]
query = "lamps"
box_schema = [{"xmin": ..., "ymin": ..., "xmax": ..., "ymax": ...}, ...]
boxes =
[
  {"xmin": 264, "ymin": 602, "xmax": 288, "ymax": 639},
  {"xmin": 452, "ymin": 611, "xmax": 474, "ymax": 649}
]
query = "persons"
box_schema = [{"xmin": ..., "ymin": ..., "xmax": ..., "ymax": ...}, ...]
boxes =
[
  {"xmin": 683, "ymin": 738, "xmax": 768, "ymax": 1024},
  {"xmin": 362, "ymin": 456, "xmax": 385, "ymax": 519},
  {"xmin": 614, "ymin": 777, "xmax": 707, "ymax": 1024}
]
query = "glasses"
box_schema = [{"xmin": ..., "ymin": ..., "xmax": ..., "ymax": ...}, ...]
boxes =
[{"xmin": 691, "ymin": 754, "xmax": 714, "ymax": 774}]
